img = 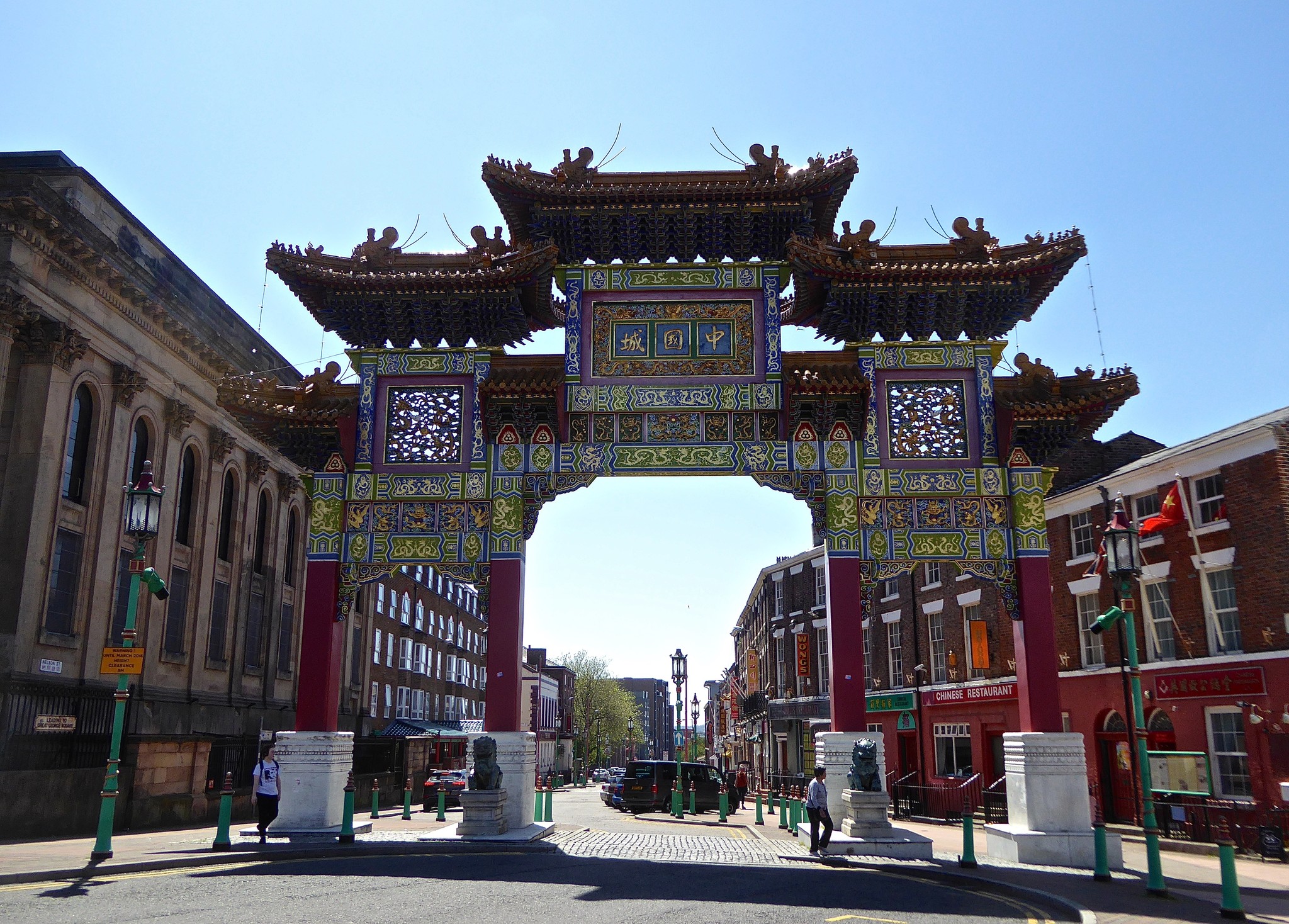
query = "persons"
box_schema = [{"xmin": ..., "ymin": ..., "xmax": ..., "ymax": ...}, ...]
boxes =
[
  {"xmin": 723, "ymin": 767, "xmax": 731, "ymax": 783},
  {"xmin": 251, "ymin": 744, "xmax": 281, "ymax": 845},
  {"xmin": 806, "ymin": 766, "xmax": 833, "ymax": 857},
  {"xmin": 735, "ymin": 767, "xmax": 749, "ymax": 809},
  {"xmin": 1089, "ymin": 787, "xmax": 1096, "ymax": 827}
]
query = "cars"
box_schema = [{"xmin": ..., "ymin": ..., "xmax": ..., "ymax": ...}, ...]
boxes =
[
  {"xmin": 600, "ymin": 767, "xmax": 630, "ymax": 812},
  {"xmin": 592, "ymin": 769, "xmax": 609, "ymax": 783}
]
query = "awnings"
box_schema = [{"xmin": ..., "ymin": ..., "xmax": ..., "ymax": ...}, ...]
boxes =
[
  {"xmin": 719, "ymin": 750, "xmax": 732, "ymax": 758},
  {"xmin": 745, "ymin": 733, "xmax": 764, "ymax": 745}
]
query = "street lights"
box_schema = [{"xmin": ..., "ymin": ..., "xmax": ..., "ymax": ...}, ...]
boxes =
[
  {"xmin": 1101, "ymin": 494, "xmax": 1168, "ymax": 898},
  {"xmin": 690, "ymin": 693, "xmax": 701, "ymax": 764},
  {"xmin": 670, "ymin": 649, "xmax": 688, "ymax": 819},
  {"xmin": 88, "ymin": 460, "xmax": 165, "ymax": 862},
  {"xmin": 597, "ymin": 733, "xmax": 602, "ymax": 769},
  {"xmin": 572, "ymin": 721, "xmax": 579, "ymax": 787},
  {"xmin": 623, "ymin": 738, "xmax": 626, "ymax": 766},
  {"xmin": 627, "ymin": 716, "xmax": 634, "ymax": 761},
  {"xmin": 554, "ymin": 711, "xmax": 562, "ymax": 790},
  {"xmin": 604, "ymin": 735, "xmax": 610, "ymax": 769},
  {"xmin": 581, "ymin": 725, "xmax": 589, "ymax": 787}
]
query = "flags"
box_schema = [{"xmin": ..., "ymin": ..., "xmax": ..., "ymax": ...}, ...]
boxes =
[
  {"xmin": 1137, "ymin": 480, "xmax": 1191, "ymax": 535},
  {"xmin": 1206, "ymin": 502, "xmax": 1227, "ymax": 523},
  {"xmin": 1082, "ymin": 536, "xmax": 1107, "ymax": 577}
]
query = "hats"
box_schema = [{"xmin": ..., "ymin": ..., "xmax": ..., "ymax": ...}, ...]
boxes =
[
  {"xmin": 261, "ymin": 743, "xmax": 275, "ymax": 757},
  {"xmin": 739, "ymin": 764, "xmax": 744, "ymax": 769}
]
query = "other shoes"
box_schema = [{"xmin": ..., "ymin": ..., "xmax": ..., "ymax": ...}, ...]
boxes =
[
  {"xmin": 259, "ymin": 836, "xmax": 266, "ymax": 843},
  {"xmin": 742, "ymin": 806, "xmax": 746, "ymax": 810},
  {"xmin": 817, "ymin": 845, "xmax": 829, "ymax": 853},
  {"xmin": 257, "ymin": 824, "xmax": 266, "ymax": 836},
  {"xmin": 809, "ymin": 849, "xmax": 820, "ymax": 857}
]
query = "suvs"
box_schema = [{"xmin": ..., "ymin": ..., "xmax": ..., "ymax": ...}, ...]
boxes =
[
  {"xmin": 620, "ymin": 760, "xmax": 741, "ymax": 817},
  {"xmin": 422, "ymin": 768, "xmax": 467, "ymax": 812}
]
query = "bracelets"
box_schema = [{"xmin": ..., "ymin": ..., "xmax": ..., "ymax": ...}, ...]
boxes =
[{"xmin": 252, "ymin": 796, "xmax": 256, "ymax": 797}]
